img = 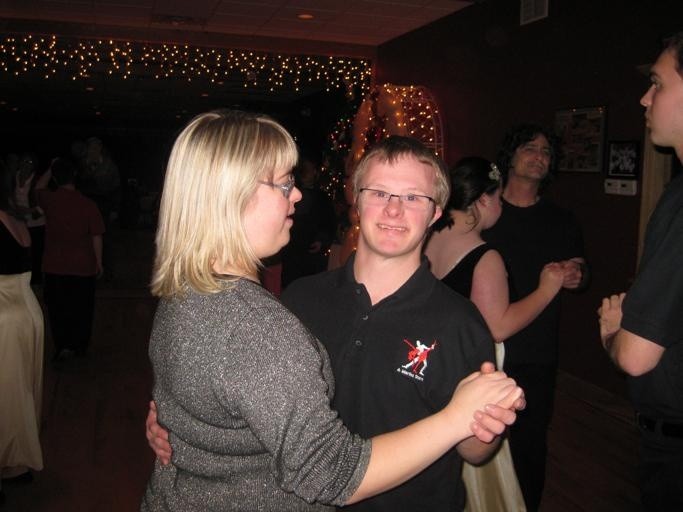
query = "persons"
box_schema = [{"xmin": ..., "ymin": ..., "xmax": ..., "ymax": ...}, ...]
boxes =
[
  {"xmin": 278, "ymin": 157, "xmax": 337, "ymax": 291},
  {"xmin": 422, "ymin": 157, "xmax": 580, "ymax": 512},
  {"xmin": 2, "ymin": 136, "xmax": 120, "ymax": 484},
  {"xmin": 480, "ymin": 127, "xmax": 587, "ymax": 512},
  {"xmin": 596, "ymin": 25, "xmax": 682, "ymax": 511}
]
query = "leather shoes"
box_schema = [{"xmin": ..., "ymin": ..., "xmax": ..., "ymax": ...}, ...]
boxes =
[{"xmin": 0, "ymin": 471, "xmax": 34, "ymax": 485}]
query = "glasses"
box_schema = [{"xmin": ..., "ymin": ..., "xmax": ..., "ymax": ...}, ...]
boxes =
[
  {"xmin": 357, "ymin": 188, "xmax": 436, "ymax": 208},
  {"xmin": 257, "ymin": 173, "xmax": 295, "ymax": 199}
]
